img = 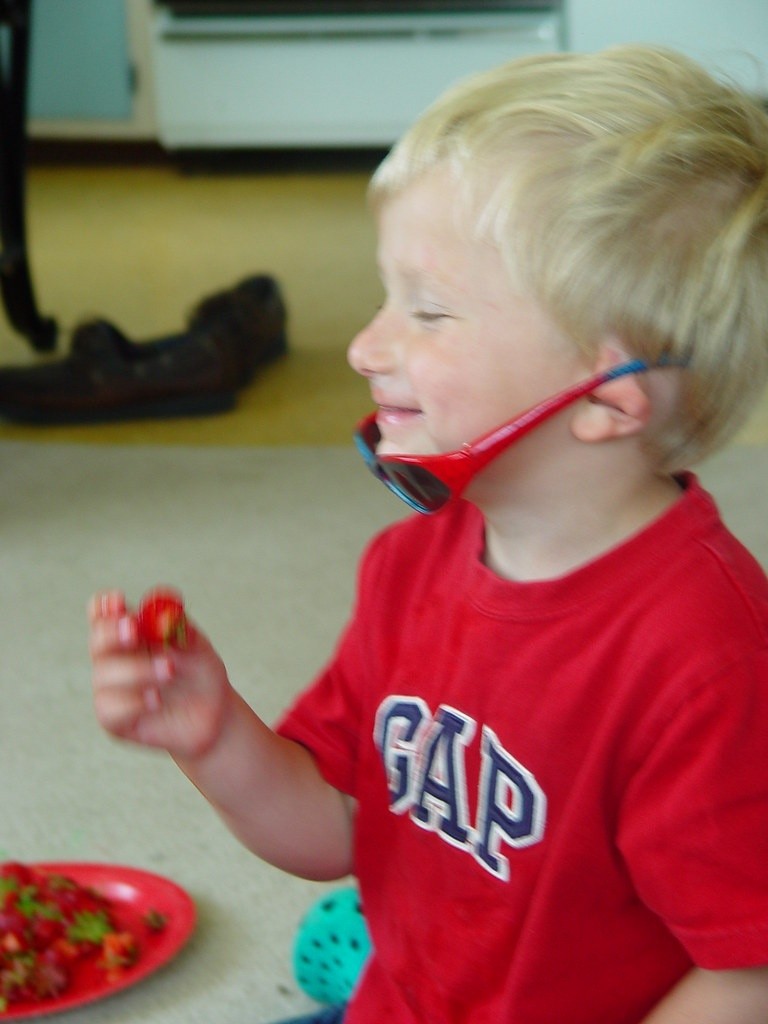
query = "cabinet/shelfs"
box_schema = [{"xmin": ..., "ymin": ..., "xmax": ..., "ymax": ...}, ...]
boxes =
[{"xmin": 150, "ymin": 1, "xmax": 561, "ymax": 176}]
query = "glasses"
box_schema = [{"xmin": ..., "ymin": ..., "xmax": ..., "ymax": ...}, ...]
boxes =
[{"xmin": 355, "ymin": 357, "xmax": 692, "ymax": 522}]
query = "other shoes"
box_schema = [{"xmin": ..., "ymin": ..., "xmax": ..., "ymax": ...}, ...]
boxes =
[
  {"xmin": 0, "ymin": 318, "xmax": 236, "ymax": 425},
  {"xmin": 190, "ymin": 276, "xmax": 286, "ymax": 389}
]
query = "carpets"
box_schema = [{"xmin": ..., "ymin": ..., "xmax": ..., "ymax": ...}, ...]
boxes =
[{"xmin": 0, "ymin": 438, "xmax": 768, "ymax": 1024}]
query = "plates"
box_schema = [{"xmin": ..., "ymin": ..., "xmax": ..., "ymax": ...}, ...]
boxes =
[{"xmin": 1, "ymin": 857, "xmax": 196, "ymax": 1021}]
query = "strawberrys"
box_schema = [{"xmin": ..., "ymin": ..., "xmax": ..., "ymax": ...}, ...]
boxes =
[
  {"xmin": 137, "ymin": 591, "xmax": 189, "ymax": 653},
  {"xmin": 0, "ymin": 861, "xmax": 140, "ymax": 1011}
]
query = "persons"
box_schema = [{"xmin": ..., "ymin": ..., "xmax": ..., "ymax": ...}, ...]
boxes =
[{"xmin": 85, "ymin": 42, "xmax": 768, "ymax": 1024}]
